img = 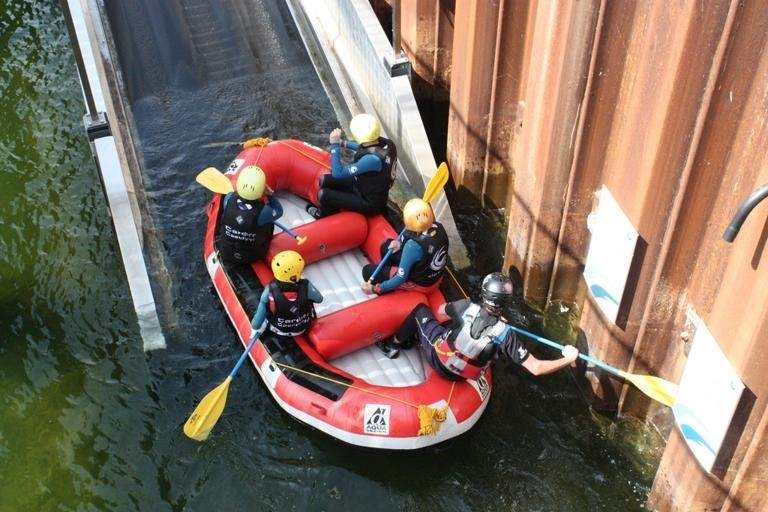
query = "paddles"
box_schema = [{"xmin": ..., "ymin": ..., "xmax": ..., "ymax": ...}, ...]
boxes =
[
  {"xmin": 510, "ymin": 325, "xmax": 679, "ymax": 407},
  {"xmin": 195, "ymin": 167, "xmax": 307, "ymax": 245},
  {"xmin": 368, "ymin": 161, "xmax": 449, "ymax": 285},
  {"xmin": 183, "ymin": 318, "xmax": 268, "ymax": 441}
]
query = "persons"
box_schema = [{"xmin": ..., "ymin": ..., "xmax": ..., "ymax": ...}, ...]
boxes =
[
  {"xmin": 376, "ymin": 271, "xmax": 579, "ymax": 381},
  {"xmin": 306, "ymin": 112, "xmax": 397, "ymax": 223},
  {"xmin": 361, "ymin": 195, "xmax": 451, "ymax": 294},
  {"xmin": 213, "ymin": 163, "xmax": 284, "ymax": 271},
  {"xmin": 249, "ymin": 250, "xmax": 324, "ymax": 342}
]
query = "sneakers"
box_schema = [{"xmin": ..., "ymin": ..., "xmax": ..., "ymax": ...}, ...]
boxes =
[
  {"xmin": 397, "ymin": 333, "xmax": 414, "ymax": 349},
  {"xmin": 306, "ymin": 203, "xmax": 321, "ymax": 219},
  {"xmin": 375, "ymin": 339, "xmax": 399, "ymax": 359}
]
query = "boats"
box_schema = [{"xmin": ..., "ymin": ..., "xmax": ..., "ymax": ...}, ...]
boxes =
[{"xmin": 203, "ymin": 137, "xmax": 494, "ymax": 451}]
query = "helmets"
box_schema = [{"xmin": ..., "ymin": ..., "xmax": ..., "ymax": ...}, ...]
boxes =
[
  {"xmin": 481, "ymin": 271, "xmax": 515, "ymax": 306},
  {"xmin": 235, "ymin": 165, "xmax": 266, "ymax": 201},
  {"xmin": 271, "ymin": 250, "xmax": 305, "ymax": 284},
  {"xmin": 403, "ymin": 198, "xmax": 434, "ymax": 232},
  {"xmin": 349, "ymin": 114, "xmax": 381, "ymax": 145}
]
